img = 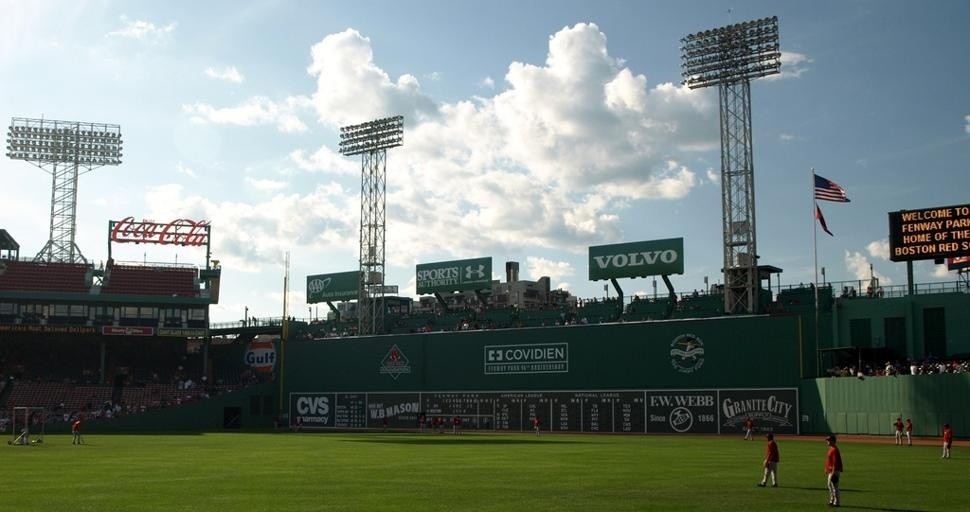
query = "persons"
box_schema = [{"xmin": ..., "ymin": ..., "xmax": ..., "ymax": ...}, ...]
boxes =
[
  {"xmin": 533, "ymin": 415, "xmax": 542, "ymax": 437},
  {"xmin": 293, "ymin": 415, "xmax": 304, "ymax": 433},
  {"xmin": 757, "ymin": 433, "xmax": 780, "ymax": 489},
  {"xmin": 419, "ymin": 413, "xmax": 463, "ymax": 434},
  {"xmin": 744, "ymin": 417, "xmax": 754, "ymax": 441},
  {"xmin": 383, "ymin": 415, "xmax": 389, "ymax": 432},
  {"xmin": 942, "ymin": 423, "xmax": 953, "ymax": 456},
  {"xmin": 893, "ymin": 417, "xmax": 904, "ymax": 445},
  {"xmin": 288, "ymin": 284, "xmax": 970, "ymax": 341},
  {"xmin": 827, "ymin": 352, "xmax": 970, "ymax": 378},
  {"xmin": 1, "ymin": 317, "xmax": 285, "ymax": 444},
  {"xmin": 824, "ymin": 435, "xmax": 843, "ymax": 507},
  {"xmin": 906, "ymin": 419, "xmax": 913, "ymax": 446}
]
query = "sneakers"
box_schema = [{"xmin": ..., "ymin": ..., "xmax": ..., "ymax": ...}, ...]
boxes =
[
  {"xmin": 827, "ymin": 502, "xmax": 839, "ymax": 507},
  {"xmin": 772, "ymin": 483, "xmax": 778, "ymax": 487},
  {"xmin": 758, "ymin": 482, "xmax": 766, "ymax": 487}
]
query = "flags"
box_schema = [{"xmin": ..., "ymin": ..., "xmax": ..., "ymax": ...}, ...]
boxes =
[
  {"xmin": 816, "ymin": 204, "xmax": 833, "ymax": 236},
  {"xmin": 815, "ymin": 174, "xmax": 850, "ymax": 202}
]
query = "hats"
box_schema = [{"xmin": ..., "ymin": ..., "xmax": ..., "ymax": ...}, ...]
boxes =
[
  {"xmin": 765, "ymin": 433, "xmax": 774, "ymax": 438},
  {"xmin": 825, "ymin": 434, "xmax": 836, "ymax": 441}
]
